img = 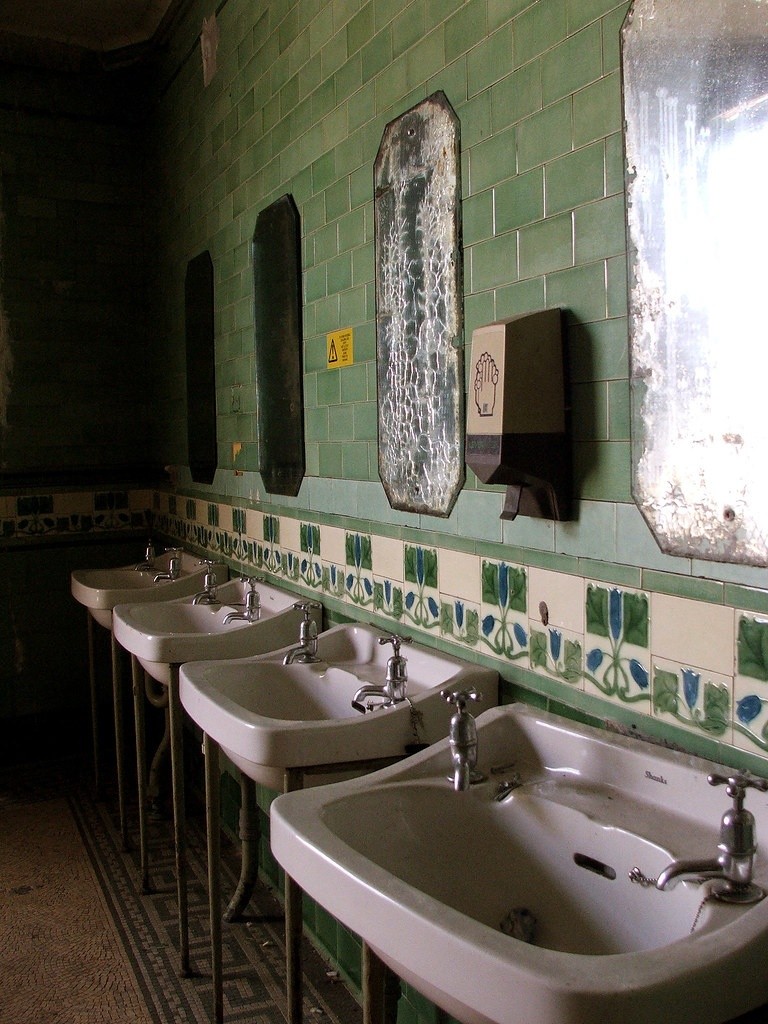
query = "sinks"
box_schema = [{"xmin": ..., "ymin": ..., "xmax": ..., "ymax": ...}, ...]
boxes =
[
  {"xmin": 111, "ymin": 591, "xmax": 258, "ymax": 663},
  {"xmin": 69, "ymin": 557, "xmax": 162, "ymax": 612},
  {"xmin": 178, "ymin": 639, "xmax": 413, "ymax": 765},
  {"xmin": 268, "ymin": 768, "xmax": 734, "ymax": 1024}
]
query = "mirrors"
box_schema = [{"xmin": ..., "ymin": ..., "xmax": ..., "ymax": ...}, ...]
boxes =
[
  {"xmin": 252, "ymin": 193, "xmax": 306, "ymax": 497},
  {"xmin": 185, "ymin": 250, "xmax": 217, "ymax": 486},
  {"xmin": 617, "ymin": 0, "xmax": 768, "ymax": 565}
]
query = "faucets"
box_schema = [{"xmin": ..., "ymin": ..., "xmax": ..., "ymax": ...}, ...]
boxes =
[
  {"xmin": 653, "ymin": 766, "xmax": 768, "ymax": 894},
  {"xmin": 352, "ymin": 633, "xmax": 413, "ymax": 702},
  {"xmin": 282, "ymin": 599, "xmax": 322, "ymax": 667},
  {"xmin": 153, "ymin": 546, "xmax": 185, "ymax": 583},
  {"xmin": 190, "ymin": 557, "xmax": 219, "ymax": 606},
  {"xmin": 222, "ymin": 574, "xmax": 265, "ymax": 626},
  {"xmin": 438, "ymin": 686, "xmax": 490, "ymax": 792},
  {"xmin": 133, "ymin": 536, "xmax": 157, "ymax": 572}
]
